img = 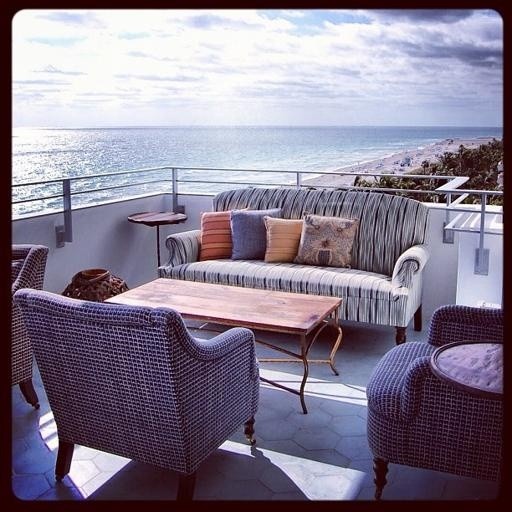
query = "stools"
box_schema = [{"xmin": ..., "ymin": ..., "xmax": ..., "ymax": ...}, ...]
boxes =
[{"xmin": 127, "ymin": 212, "xmax": 186, "ymax": 273}]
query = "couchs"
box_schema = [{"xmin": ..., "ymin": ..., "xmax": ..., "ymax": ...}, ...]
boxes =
[
  {"xmin": 158, "ymin": 188, "xmax": 432, "ymax": 345},
  {"xmin": 366, "ymin": 304, "xmax": 503, "ymax": 499},
  {"xmin": 12, "ymin": 243, "xmax": 49, "ymax": 411},
  {"xmin": 14, "ymin": 288, "xmax": 260, "ymax": 499}
]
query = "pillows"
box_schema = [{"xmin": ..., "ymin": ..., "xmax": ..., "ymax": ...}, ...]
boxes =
[
  {"xmin": 199, "ymin": 208, "xmax": 248, "ymax": 261},
  {"xmin": 263, "ymin": 215, "xmax": 303, "ymax": 263},
  {"xmin": 231, "ymin": 208, "xmax": 285, "ymax": 260},
  {"xmin": 293, "ymin": 211, "xmax": 360, "ymax": 269}
]
